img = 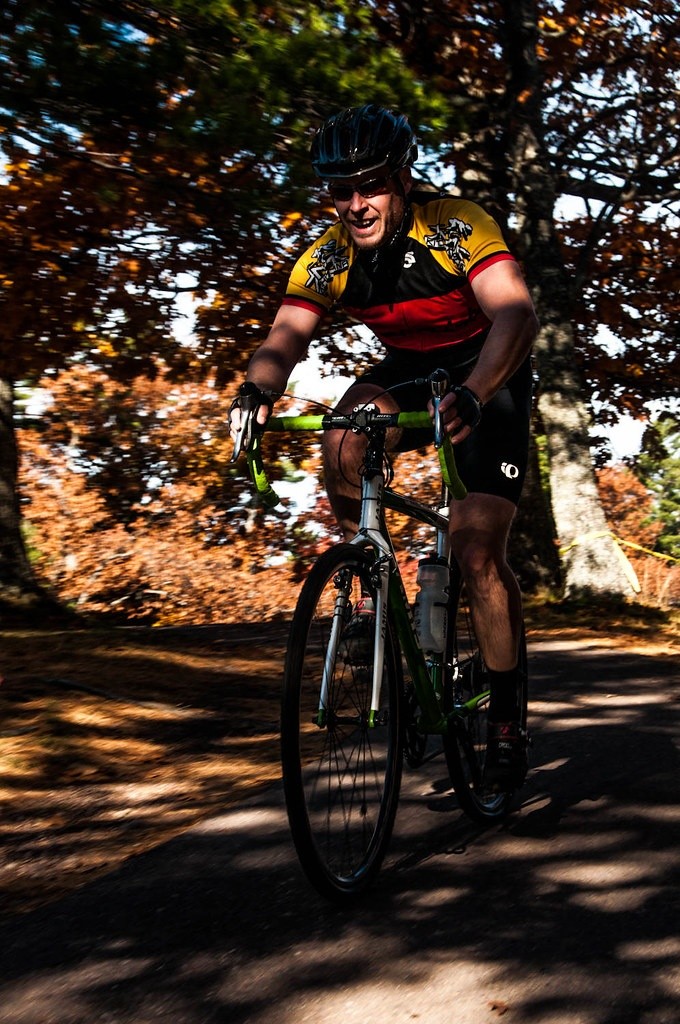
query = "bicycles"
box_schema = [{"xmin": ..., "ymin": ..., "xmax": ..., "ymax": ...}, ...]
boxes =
[{"xmin": 231, "ymin": 367, "xmax": 532, "ymax": 909}]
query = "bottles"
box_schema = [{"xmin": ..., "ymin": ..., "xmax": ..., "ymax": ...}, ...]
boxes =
[{"xmin": 413, "ymin": 550, "xmax": 450, "ymax": 651}]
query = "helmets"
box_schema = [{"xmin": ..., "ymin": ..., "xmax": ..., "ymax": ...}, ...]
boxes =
[{"xmin": 311, "ymin": 103, "xmax": 416, "ymax": 184}]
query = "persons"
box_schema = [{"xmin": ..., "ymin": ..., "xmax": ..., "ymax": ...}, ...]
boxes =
[{"xmin": 228, "ymin": 105, "xmax": 540, "ymax": 817}]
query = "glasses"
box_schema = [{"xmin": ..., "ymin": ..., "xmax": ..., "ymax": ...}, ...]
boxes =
[{"xmin": 330, "ymin": 174, "xmax": 400, "ymax": 199}]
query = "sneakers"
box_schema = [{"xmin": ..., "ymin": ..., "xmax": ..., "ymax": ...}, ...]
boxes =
[
  {"xmin": 343, "ymin": 584, "xmax": 378, "ymax": 637},
  {"xmin": 477, "ymin": 722, "xmax": 532, "ymax": 818}
]
